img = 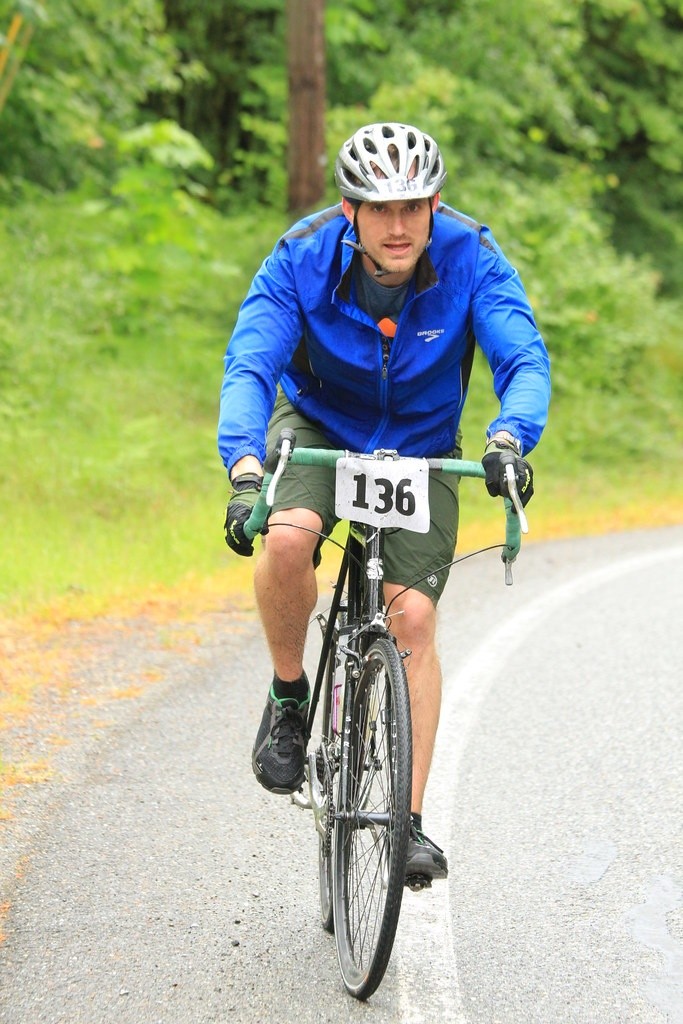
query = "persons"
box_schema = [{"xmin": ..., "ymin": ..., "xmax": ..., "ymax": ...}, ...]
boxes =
[{"xmin": 217, "ymin": 121, "xmax": 551, "ymax": 880}]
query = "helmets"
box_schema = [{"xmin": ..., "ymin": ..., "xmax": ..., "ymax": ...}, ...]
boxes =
[{"xmin": 334, "ymin": 123, "xmax": 447, "ymax": 203}]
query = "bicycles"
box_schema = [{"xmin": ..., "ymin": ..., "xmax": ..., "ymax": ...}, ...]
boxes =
[{"xmin": 236, "ymin": 425, "xmax": 531, "ymax": 1000}]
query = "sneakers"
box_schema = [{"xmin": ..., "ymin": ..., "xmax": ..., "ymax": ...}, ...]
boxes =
[
  {"xmin": 403, "ymin": 825, "xmax": 448, "ymax": 879},
  {"xmin": 251, "ymin": 682, "xmax": 312, "ymax": 796}
]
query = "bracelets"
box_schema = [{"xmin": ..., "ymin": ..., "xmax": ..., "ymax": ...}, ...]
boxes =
[{"xmin": 485, "ymin": 432, "xmax": 521, "ymax": 457}]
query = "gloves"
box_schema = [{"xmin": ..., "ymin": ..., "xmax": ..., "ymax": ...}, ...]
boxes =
[
  {"xmin": 223, "ymin": 472, "xmax": 272, "ymax": 557},
  {"xmin": 481, "ymin": 441, "xmax": 534, "ymax": 514}
]
